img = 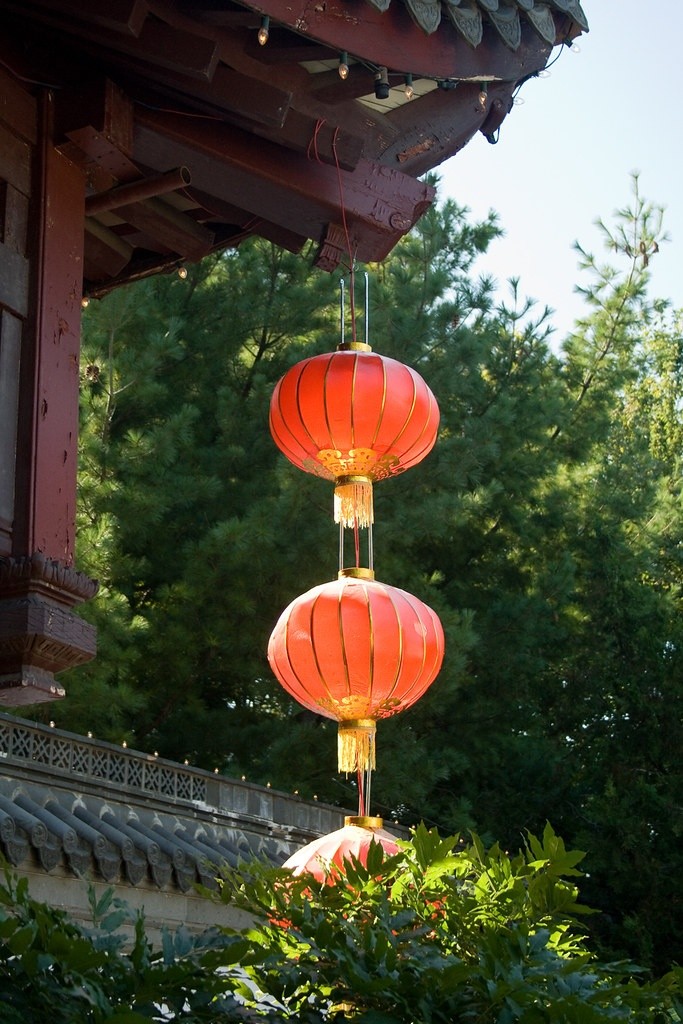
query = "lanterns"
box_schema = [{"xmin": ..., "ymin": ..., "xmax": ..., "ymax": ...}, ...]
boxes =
[
  {"xmin": 267, "ymin": 568, "xmax": 444, "ymax": 774},
  {"xmin": 269, "ymin": 817, "xmax": 446, "ymax": 955},
  {"xmin": 269, "ymin": 343, "xmax": 440, "ymax": 529}
]
